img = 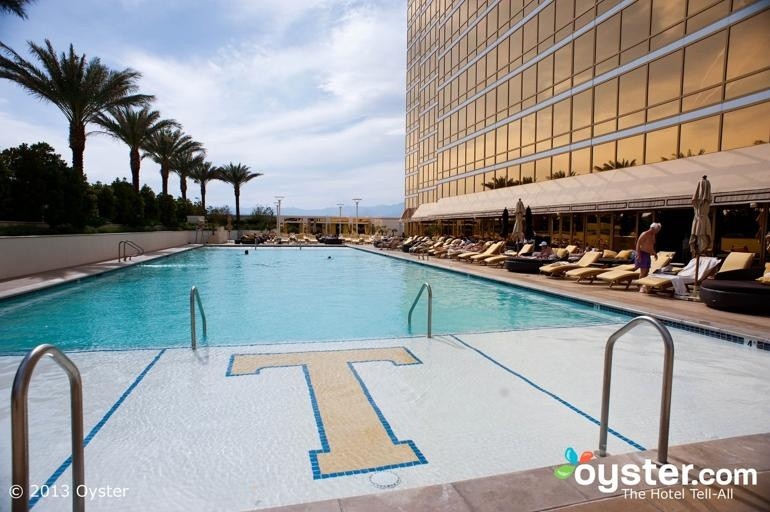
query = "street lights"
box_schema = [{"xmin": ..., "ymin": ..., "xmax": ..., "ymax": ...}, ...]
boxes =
[
  {"xmin": 273, "ymin": 195, "xmax": 285, "ymax": 235},
  {"xmin": 336, "ymin": 202, "xmax": 345, "ymax": 234},
  {"xmin": 351, "ymin": 198, "xmax": 362, "ymax": 233}
]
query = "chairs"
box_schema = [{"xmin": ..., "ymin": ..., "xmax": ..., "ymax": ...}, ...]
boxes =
[{"xmin": 234, "ymin": 230, "xmax": 770, "ymax": 318}]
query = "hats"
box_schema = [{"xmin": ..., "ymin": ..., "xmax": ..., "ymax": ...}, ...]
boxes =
[{"xmin": 539, "ymin": 241, "xmax": 547, "ymax": 246}]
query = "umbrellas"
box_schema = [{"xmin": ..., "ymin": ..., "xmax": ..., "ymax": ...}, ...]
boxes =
[
  {"xmin": 511, "ymin": 197, "xmax": 526, "ymax": 258},
  {"xmin": 688, "ymin": 176, "xmax": 714, "ymax": 290},
  {"xmin": 500, "ymin": 207, "xmax": 510, "ymax": 242},
  {"xmin": 523, "ymin": 205, "xmax": 534, "ymax": 245}
]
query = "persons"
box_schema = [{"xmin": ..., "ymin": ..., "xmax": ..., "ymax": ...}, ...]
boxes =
[
  {"xmin": 635, "ymin": 222, "xmax": 662, "ymax": 292},
  {"xmin": 533, "ymin": 241, "xmax": 555, "ymax": 260},
  {"xmin": 378, "ymin": 235, "xmax": 486, "ymax": 261},
  {"xmin": 584, "ymin": 246, "xmax": 592, "ymax": 253}
]
